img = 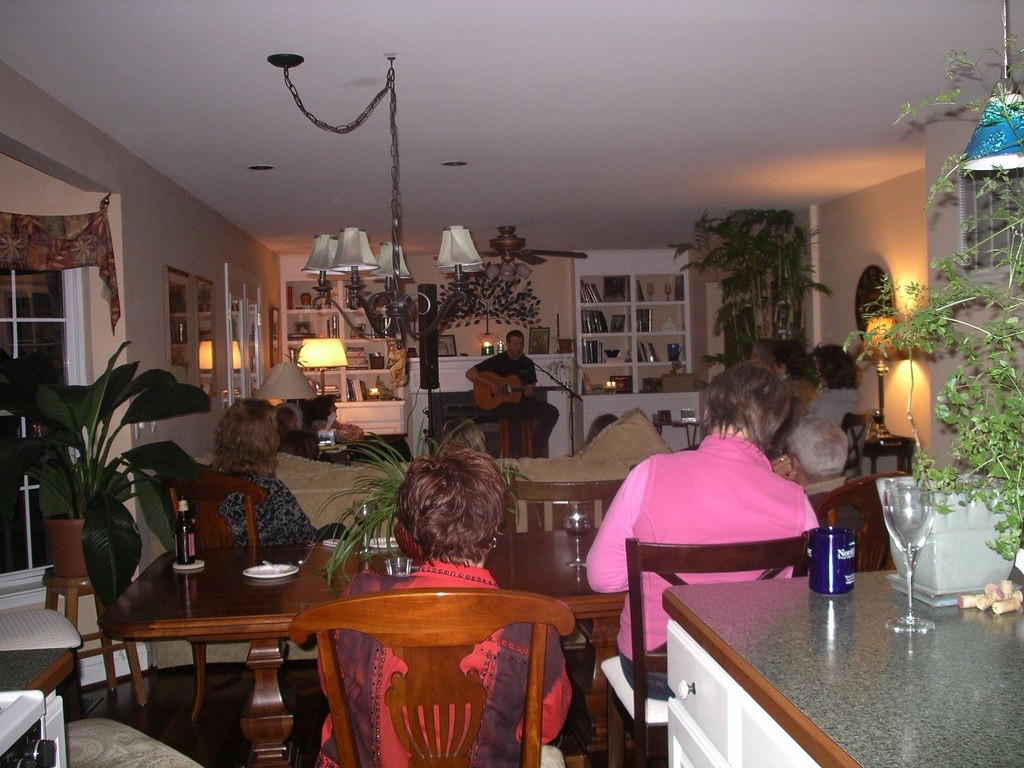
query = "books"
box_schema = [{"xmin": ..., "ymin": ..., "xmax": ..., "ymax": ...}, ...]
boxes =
[
  {"xmin": 637, "ymin": 309, "xmax": 653, "ymax": 332},
  {"xmin": 581, "ymin": 309, "xmax": 608, "ymax": 333},
  {"xmin": 288, "ymin": 285, "xmax": 387, "ymax": 402},
  {"xmin": 578, "ymin": 280, "xmax": 602, "ymax": 302},
  {"xmin": 637, "ymin": 342, "xmax": 659, "ymax": 362},
  {"xmin": 581, "ymin": 337, "xmax": 603, "ymax": 364}
]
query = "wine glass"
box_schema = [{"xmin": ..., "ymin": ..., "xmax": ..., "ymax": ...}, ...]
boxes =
[
  {"xmin": 883, "ymin": 479, "xmax": 936, "ymax": 635},
  {"xmin": 664, "ymin": 282, "xmax": 671, "ymax": 301},
  {"xmin": 872, "ymin": 408, "xmax": 887, "ymax": 438},
  {"xmin": 353, "ymin": 500, "xmax": 377, "ymax": 556},
  {"xmin": 562, "ymin": 499, "xmax": 590, "ymax": 567},
  {"xmin": 646, "ymin": 283, "xmax": 654, "ymax": 302}
]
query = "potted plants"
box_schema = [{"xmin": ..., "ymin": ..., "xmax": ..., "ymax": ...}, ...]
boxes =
[{"xmin": 0, "ymin": 342, "xmax": 212, "ymax": 611}]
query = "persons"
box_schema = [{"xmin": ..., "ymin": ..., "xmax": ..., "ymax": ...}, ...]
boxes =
[
  {"xmin": 314, "ymin": 443, "xmax": 570, "ymax": 768},
  {"xmin": 580, "ymin": 414, "xmax": 619, "ymax": 448},
  {"xmin": 752, "ymin": 340, "xmax": 811, "ymax": 385},
  {"xmin": 586, "ymin": 360, "xmax": 819, "ymax": 702},
  {"xmin": 807, "ymin": 344, "xmax": 868, "ymax": 479},
  {"xmin": 767, "ymin": 412, "xmax": 849, "ymax": 488},
  {"xmin": 465, "ymin": 330, "xmax": 559, "ymax": 458},
  {"xmin": 279, "ymin": 395, "xmax": 337, "ymax": 461},
  {"xmin": 188, "ymin": 396, "xmax": 351, "ymax": 551}
]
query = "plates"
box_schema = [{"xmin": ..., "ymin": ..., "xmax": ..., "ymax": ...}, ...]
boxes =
[
  {"xmin": 363, "ymin": 537, "xmax": 399, "ymax": 548},
  {"xmin": 242, "ymin": 564, "xmax": 299, "ymax": 579}
]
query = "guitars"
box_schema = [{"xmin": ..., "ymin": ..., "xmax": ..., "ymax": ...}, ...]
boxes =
[{"xmin": 473, "ymin": 370, "xmax": 572, "ymax": 411}]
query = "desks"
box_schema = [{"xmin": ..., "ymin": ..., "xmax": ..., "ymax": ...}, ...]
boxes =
[
  {"xmin": 100, "ymin": 534, "xmax": 621, "ymax": 768},
  {"xmin": 863, "ymin": 437, "xmax": 915, "ymax": 475},
  {"xmin": 0, "ymin": 617, "xmax": 73, "ymax": 695}
]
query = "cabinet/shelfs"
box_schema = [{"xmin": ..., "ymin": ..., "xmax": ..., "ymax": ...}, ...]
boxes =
[
  {"xmin": 279, "ymin": 252, "xmax": 403, "ymax": 400},
  {"xmin": 574, "ymin": 246, "xmax": 694, "ymax": 392},
  {"xmin": 662, "ymin": 583, "xmax": 856, "ymax": 768}
]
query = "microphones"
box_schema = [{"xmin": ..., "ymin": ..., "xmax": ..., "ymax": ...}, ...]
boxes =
[{"xmin": 519, "ymin": 353, "xmax": 529, "ymax": 362}]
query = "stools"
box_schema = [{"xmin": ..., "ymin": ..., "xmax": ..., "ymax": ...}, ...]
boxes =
[{"xmin": 40, "ymin": 569, "xmax": 151, "ymax": 706}]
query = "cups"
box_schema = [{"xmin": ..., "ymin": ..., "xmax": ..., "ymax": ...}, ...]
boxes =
[
  {"xmin": 383, "ymin": 557, "xmax": 413, "ymax": 575},
  {"xmin": 497, "ymin": 340, "xmax": 505, "ymax": 353},
  {"xmin": 300, "ymin": 293, "xmax": 311, "ymax": 305},
  {"xmin": 808, "ymin": 527, "xmax": 856, "ymax": 596},
  {"xmin": 667, "ymin": 344, "xmax": 683, "ymax": 361}
]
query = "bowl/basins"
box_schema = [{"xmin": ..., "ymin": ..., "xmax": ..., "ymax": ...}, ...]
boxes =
[{"xmin": 604, "ymin": 349, "xmax": 620, "ymax": 358}]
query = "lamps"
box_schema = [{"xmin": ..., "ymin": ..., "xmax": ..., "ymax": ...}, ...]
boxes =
[
  {"xmin": 297, "ymin": 339, "xmax": 348, "ymax": 395},
  {"xmin": 486, "ymin": 254, "xmax": 532, "ymax": 282},
  {"xmin": 864, "ymin": 316, "xmax": 902, "ymax": 442},
  {"xmin": 266, "ymin": 53, "xmax": 484, "ymax": 350},
  {"xmin": 253, "ymin": 362, "xmax": 319, "ymax": 404},
  {"xmin": 959, "ymin": 0, "xmax": 1024, "ymax": 169}
]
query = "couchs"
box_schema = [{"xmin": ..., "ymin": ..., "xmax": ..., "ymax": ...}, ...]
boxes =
[{"xmin": 192, "ymin": 406, "xmax": 674, "ymax": 540}]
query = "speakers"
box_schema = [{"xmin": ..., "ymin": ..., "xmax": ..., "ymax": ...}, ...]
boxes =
[{"xmin": 418, "ymin": 284, "xmax": 440, "ymax": 389}]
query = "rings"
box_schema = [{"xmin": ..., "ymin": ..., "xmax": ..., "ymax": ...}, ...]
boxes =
[{"xmin": 780, "ymin": 455, "xmax": 784, "ymax": 460}]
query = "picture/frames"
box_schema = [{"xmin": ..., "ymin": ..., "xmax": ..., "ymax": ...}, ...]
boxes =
[
  {"xmin": 437, "ymin": 335, "xmax": 457, "ymax": 357},
  {"xmin": 529, "ymin": 327, "xmax": 550, "ymax": 354}
]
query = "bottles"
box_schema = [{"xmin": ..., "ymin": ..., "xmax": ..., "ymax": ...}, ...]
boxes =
[{"xmin": 174, "ymin": 495, "xmax": 195, "ymax": 565}]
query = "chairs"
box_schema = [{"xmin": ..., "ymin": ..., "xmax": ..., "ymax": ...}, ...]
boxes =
[{"xmin": 170, "ymin": 412, "xmax": 911, "ymax": 768}]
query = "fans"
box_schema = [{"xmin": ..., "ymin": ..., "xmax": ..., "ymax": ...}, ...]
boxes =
[{"xmin": 479, "ymin": 225, "xmax": 587, "ymax": 265}]
